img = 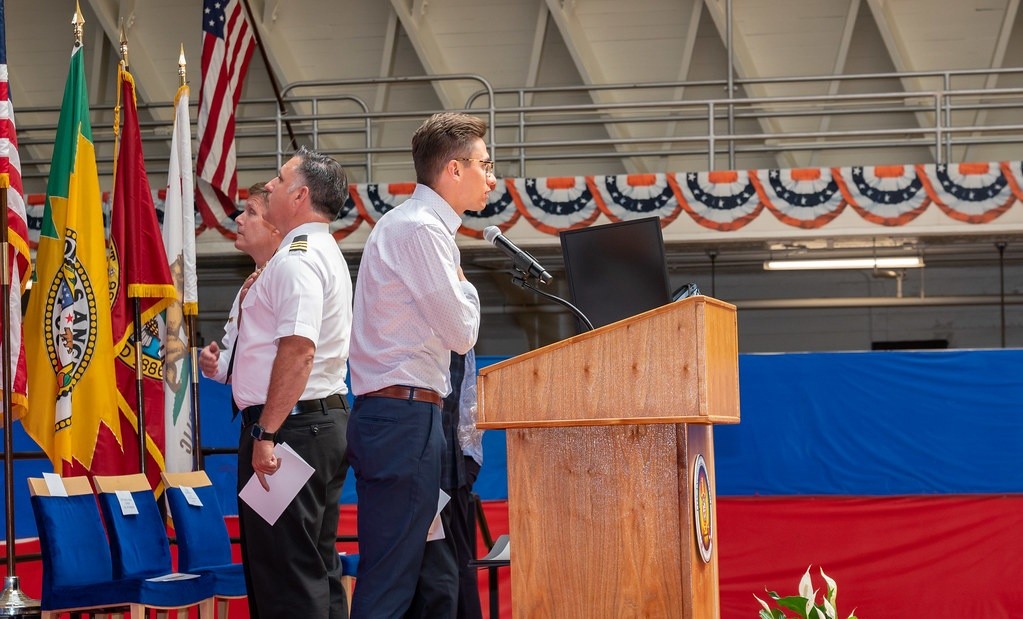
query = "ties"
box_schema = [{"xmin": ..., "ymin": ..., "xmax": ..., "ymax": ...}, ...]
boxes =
[{"xmin": 225, "ymin": 247, "xmax": 278, "ymax": 423}]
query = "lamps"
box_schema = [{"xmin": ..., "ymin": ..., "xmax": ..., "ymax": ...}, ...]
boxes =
[
  {"xmin": 762, "ymin": 256, "xmax": 927, "ymax": 271},
  {"xmin": 872, "ymin": 236, "xmax": 898, "ymax": 279}
]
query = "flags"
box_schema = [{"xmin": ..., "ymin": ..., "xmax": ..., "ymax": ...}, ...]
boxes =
[
  {"xmin": 195, "ymin": 0, "xmax": 259, "ymax": 240},
  {"xmin": 0, "ymin": 0, "xmax": 198, "ymax": 544}
]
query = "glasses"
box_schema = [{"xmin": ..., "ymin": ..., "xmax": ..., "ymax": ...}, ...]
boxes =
[{"xmin": 447, "ymin": 157, "xmax": 495, "ymax": 178}]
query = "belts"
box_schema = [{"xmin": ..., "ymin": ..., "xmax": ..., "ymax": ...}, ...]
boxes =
[
  {"xmin": 241, "ymin": 394, "xmax": 350, "ymax": 428},
  {"xmin": 355, "ymin": 385, "xmax": 445, "ymax": 409}
]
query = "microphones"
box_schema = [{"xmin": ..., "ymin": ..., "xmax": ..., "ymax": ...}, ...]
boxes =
[{"xmin": 483, "ymin": 226, "xmax": 553, "ymax": 285}]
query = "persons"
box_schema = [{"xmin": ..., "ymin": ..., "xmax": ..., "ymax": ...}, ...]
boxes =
[
  {"xmin": 345, "ymin": 112, "xmax": 496, "ymax": 619},
  {"xmin": 199, "ymin": 147, "xmax": 352, "ymax": 619}
]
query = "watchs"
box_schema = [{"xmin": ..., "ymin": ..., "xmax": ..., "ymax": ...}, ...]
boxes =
[{"xmin": 250, "ymin": 423, "xmax": 274, "ymax": 441}]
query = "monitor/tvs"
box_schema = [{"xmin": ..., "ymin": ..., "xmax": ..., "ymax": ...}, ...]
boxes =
[{"xmin": 558, "ymin": 216, "xmax": 672, "ymax": 334}]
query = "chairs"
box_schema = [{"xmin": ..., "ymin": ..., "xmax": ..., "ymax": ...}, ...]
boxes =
[{"xmin": 26, "ymin": 469, "xmax": 361, "ymax": 619}]
